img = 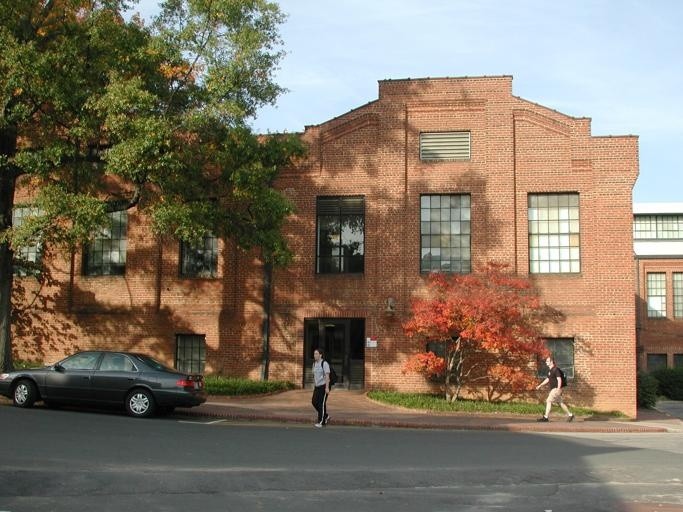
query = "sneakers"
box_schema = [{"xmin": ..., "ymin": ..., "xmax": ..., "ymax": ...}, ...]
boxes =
[
  {"xmin": 567, "ymin": 414, "xmax": 575, "ymax": 423},
  {"xmin": 314, "ymin": 415, "xmax": 329, "ymax": 428},
  {"xmin": 536, "ymin": 416, "xmax": 548, "ymax": 422}
]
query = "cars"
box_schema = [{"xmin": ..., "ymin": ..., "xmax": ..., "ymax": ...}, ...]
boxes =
[{"xmin": 0, "ymin": 351, "xmax": 207, "ymax": 417}]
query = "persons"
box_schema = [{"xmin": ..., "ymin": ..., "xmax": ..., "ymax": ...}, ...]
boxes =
[
  {"xmin": 534, "ymin": 356, "xmax": 574, "ymax": 423},
  {"xmin": 309, "ymin": 348, "xmax": 334, "ymax": 429}
]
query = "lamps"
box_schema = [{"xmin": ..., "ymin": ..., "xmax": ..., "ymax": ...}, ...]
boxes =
[{"xmin": 383, "ymin": 298, "xmax": 396, "ymax": 316}]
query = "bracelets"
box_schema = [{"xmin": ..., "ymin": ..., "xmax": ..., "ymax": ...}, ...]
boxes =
[{"xmin": 540, "ymin": 384, "xmax": 542, "ymax": 386}]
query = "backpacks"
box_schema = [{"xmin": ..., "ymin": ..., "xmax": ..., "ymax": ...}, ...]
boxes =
[
  {"xmin": 321, "ymin": 359, "xmax": 338, "ymax": 385},
  {"xmin": 561, "ymin": 371, "xmax": 567, "ymax": 386}
]
